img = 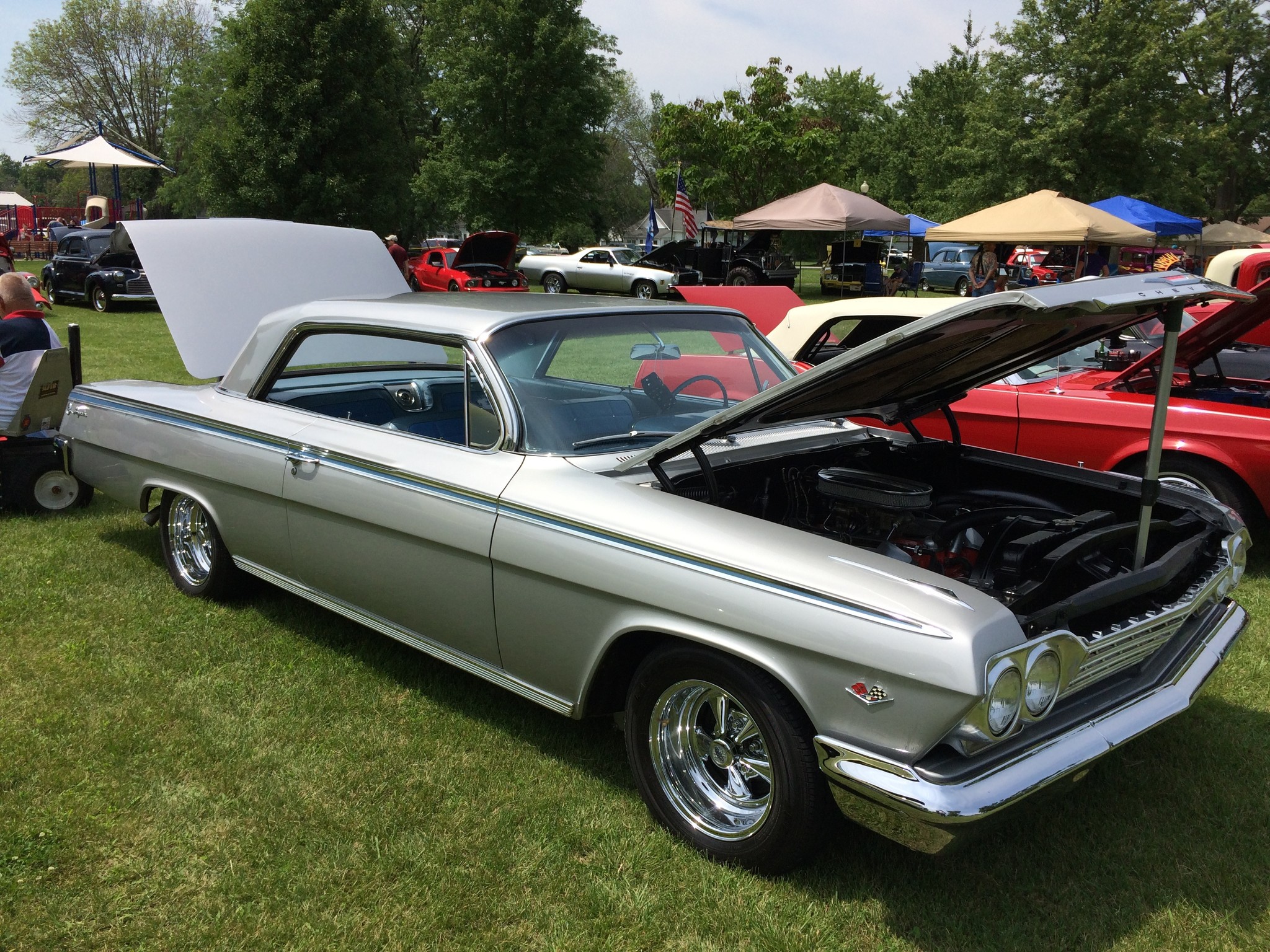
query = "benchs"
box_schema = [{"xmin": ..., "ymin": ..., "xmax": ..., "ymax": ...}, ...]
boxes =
[{"xmin": 6, "ymin": 240, "xmax": 58, "ymax": 260}]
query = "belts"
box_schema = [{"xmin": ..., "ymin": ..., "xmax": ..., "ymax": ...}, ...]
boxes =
[{"xmin": 975, "ymin": 276, "xmax": 994, "ymax": 281}]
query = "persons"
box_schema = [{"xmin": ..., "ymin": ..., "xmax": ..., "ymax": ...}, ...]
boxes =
[
  {"xmin": 1075, "ymin": 243, "xmax": 1110, "ymax": 280},
  {"xmin": 884, "ymin": 265, "xmax": 909, "ymax": 297},
  {"xmin": 17, "ymin": 223, "xmax": 34, "ymax": 261},
  {"xmin": 70, "ymin": 220, "xmax": 76, "ymax": 225},
  {"xmin": 385, "ymin": 234, "xmax": 409, "ymax": 282},
  {"xmin": 46, "ymin": 217, "xmax": 64, "ymax": 241},
  {"xmin": 969, "ymin": 241, "xmax": 997, "ymax": 298},
  {"xmin": 0, "ymin": 272, "xmax": 63, "ymax": 436}
]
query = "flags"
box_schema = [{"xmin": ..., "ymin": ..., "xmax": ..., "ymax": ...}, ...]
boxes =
[
  {"xmin": 645, "ymin": 203, "xmax": 659, "ymax": 254},
  {"xmin": 675, "ymin": 169, "xmax": 699, "ymax": 239}
]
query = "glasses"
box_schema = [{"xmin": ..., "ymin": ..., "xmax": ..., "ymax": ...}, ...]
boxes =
[{"xmin": 896, "ymin": 269, "xmax": 900, "ymax": 271}]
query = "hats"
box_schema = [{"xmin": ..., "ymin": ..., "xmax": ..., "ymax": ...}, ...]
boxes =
[
  {"xmin": 385, "ymin": 235, "xmax": 398, "ymax": 242},
  {"xmin": 894, "ymin": 265, "xmax": 900, "ymax": 270}
]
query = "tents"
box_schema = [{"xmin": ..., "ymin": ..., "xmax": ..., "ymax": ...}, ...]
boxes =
[
  {"xmin": 858, "ymin": 213, "xmax": 942, "ymax": 275},
  {"xmin": 1159, "ymin": 218, "xmax": 1270, "ymax": 274},
  {"xmin": 1088, "ymin": 196, "xmax": 1203, "ymax": 277},
  {"xmin": 924, "ymin": 189, "xmax": 1156, "ymax": 281},
  {"xmin": 726, "ymin": 183, "xmax": 910, "ymax": 299}
]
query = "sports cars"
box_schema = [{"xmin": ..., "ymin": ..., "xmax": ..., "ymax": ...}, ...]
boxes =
[{"xmin": 633, "ymin": 249, "xmax": 1270, "ymax": 532}]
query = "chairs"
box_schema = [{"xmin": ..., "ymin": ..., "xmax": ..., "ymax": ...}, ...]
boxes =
[
  {"xmin": 898, "ymin": 263, "xmax": 925, "ymax": 297},
  {"xmin": 287, "ymin": 372, "xmax": 636, "ymax": 460}
]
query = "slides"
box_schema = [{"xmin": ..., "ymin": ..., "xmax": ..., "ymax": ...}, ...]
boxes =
[{"xmin": 79, "ymin": 216, "xmax": 107, "ymax": 229}]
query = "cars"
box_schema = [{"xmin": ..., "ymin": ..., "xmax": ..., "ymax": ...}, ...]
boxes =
[
  {"xmin": 820, "ymin": 241, "xmax": 911, "ymax": 292},
  {"xmin": 1118, "ymin": 246, "xmax": 1189, "ymax": 276},
  {"xmin": 912, "ymin": 246, "xmax": 1010, "ymax": 296},
  {"xmin": 0, "ymin": 233, "xmax": 54, "ymax": 312},
  {"xmin": 518, "ymin": 238, "xmax": 707, "ymax": 300},
  {"xmin": 515, "ymin": 242, "xmax": 571, "ymax": 261},
  {"xmin": 418, "ymin": 238, "xmax": 461, "ymax": 252},
  {"xmin": 55, "ymin": 219, "xmax": 1254, "ymax": 866},
  {"xmin": 407, "ymin": 230, "xmax": 531, "ymax": 293},
  {"xmin": 1006, "ymin": 246, "xmax": 1061, "ymax": 285},
  {"xmin": 37, "ymin": 226, "xmax": 160, "ymax": 312},
  {"xmin": 680, "ymin": 225, "xmax": 799, "ymax": 291}
]
